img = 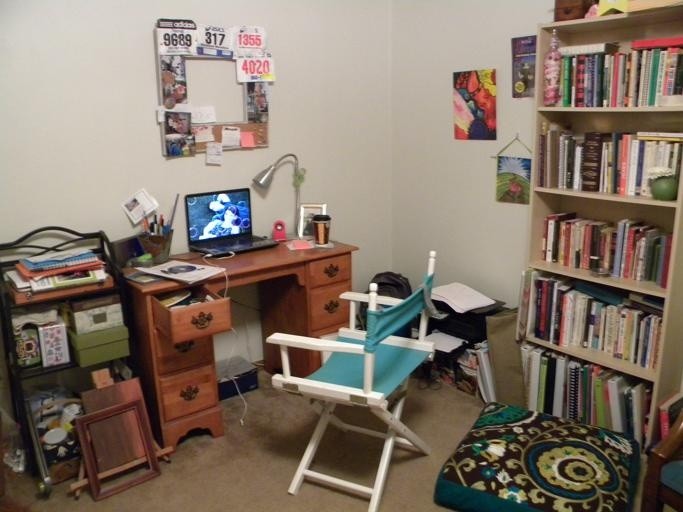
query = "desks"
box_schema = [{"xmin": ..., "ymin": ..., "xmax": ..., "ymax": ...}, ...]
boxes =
[{"xmin": 126, "ymin": 237, "xmax": 359, "ymax": 453}]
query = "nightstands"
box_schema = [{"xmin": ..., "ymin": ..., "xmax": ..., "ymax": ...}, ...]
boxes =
[{"xmin": 67, "ymin": 294, "xmax": 123, "ymax": 336}]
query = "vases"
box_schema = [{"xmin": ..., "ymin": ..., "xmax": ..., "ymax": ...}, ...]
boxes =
[{"xmin": 650, "ymin": 177, "xmax": 678, "ymax": 200}]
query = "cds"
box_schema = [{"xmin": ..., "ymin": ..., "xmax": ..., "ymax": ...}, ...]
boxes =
[{"xmin": 168, "ymin": 265, "xmax": 196, "ymax": 273}]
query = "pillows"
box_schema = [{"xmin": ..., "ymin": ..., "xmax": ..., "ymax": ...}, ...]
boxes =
[{"xmin": 434, "ymin": 400, "xmax": 641, "ymax": 512}]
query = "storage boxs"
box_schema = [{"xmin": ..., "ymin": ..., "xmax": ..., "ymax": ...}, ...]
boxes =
[
  {"xmin": 219, "ymin": 370, "xmax": 258, "ymax": 401},
  {"xmin": 69, "ymin": 326, "xmax": 132, "ymax": 369}
]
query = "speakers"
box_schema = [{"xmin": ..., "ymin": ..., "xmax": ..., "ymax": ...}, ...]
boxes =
[{"xmin": 272, "ymin": 220, "xmax": 287, "ymax": 241}]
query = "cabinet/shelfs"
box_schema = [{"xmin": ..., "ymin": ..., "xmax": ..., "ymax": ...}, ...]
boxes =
[
  {"xmin": 525, "ymin": 2, "xmax": 682, "ymax": 464},
  {"xmin": 0, "ymin": 226, "xmax": 139, "ymax": 477}
]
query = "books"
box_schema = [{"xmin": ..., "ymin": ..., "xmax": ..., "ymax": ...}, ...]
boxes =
[
  {"xmin": 541, "ymin": 212, "xmax": 672, "ymax": 289},
  {"xmin": 538, "ymin": 122, "xmax": 682, "ymax": 198},
  {"xmin": 1, "ymin": 246, "xmax": 108, "ymax": 294},
  {"xmin": 520, "ymin": 269, "xmax": 665, "ymax": 370},
  {"xmin": 551, "ymin": 37, "xmax": 682, "ymax": 107},
  {"xmin": 521, "ymin": 344, "xmax": 682, "ymax": 443}
]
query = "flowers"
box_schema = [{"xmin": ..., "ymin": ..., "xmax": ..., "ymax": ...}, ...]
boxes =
[{"xmin": 647, "ymin": 166, "xmax": 676, "ymax": 179}]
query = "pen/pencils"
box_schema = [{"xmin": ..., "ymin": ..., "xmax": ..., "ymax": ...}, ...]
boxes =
[{"xmin": 143, "ymin": 194, "xmax": 179, "ymax": 237}]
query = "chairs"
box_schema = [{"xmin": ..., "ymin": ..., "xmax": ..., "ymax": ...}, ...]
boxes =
[
  {"xmin": 266, "ymin": 250, "xmax": 436, "ymax": 512},
  {"xmin": 641, "ymin": 408, "xmax": 682, "ymax": 512}
]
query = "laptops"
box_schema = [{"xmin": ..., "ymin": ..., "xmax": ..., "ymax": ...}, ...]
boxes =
[{"xmin": 185, "ymin": 187, "xmax": 280, "ymax": 257}]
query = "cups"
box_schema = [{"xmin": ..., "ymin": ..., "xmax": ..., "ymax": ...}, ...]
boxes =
[{"xmin": 311, "ymin": 215, "xmax": 331, "ymax": 245}]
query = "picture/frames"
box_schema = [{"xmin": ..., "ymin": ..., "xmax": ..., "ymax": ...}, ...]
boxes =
[
  {"xmin": 75, "ymin": 399, "xmax": 161, "ymax": 501},
  {"xmin": 299, "ymin": 203, "xmax": 326, "ymax": 239}
]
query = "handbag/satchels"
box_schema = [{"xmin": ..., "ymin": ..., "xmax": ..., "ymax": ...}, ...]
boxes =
[{"xmin": 358, "ymin": 271, "xmax": 412, "ymax": 332}]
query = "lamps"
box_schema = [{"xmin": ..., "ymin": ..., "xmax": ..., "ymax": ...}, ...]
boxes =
[{"xmin": 252, "ymin": 154, "xmax": 300, "ymax": 240}]
